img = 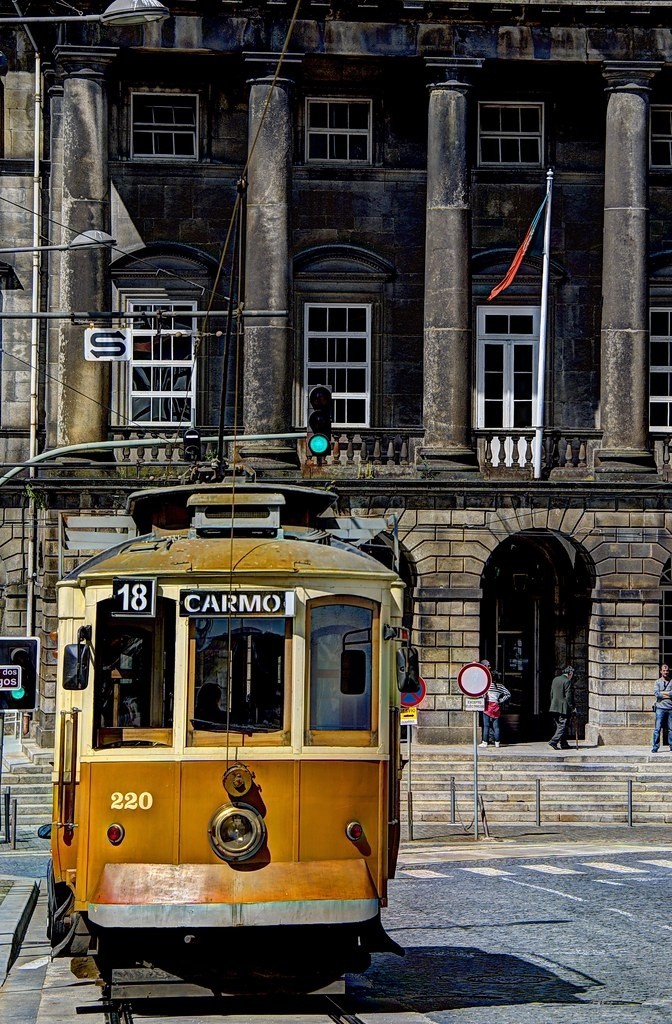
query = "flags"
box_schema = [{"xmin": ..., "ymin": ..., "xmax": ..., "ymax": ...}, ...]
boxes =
[{"xmin": 488, "ymin": 178, "xmax": 552, "ymax": 300}]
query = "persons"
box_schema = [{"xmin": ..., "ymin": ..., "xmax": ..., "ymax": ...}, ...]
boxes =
[
  {"xmin": 547, "ymin": 666, "xmax": 578, "ymax": 750},
  {"xmin": 651, "ymin": 663, "xmax": 672, "ymax": 753},
  {"xmin": 478, "ymin": 660, "xmax": 511, "ymax": 748},
  {"xmin": 194, "ymin": 683, "xmax": 231, "ymax": 724}
]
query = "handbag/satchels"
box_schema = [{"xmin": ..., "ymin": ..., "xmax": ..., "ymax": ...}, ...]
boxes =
[{"xmin": 652, "ymin": 703, "xmax": 657, "ymax": 712}]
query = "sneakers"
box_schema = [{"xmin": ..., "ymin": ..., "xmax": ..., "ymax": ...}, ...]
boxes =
[
  {"xmin": 495, "ymin": 741, "xmax": 500, "ymax": 748},
  {"xmin": 478, "ymin": 741, "xmax": 488, "ymax": 748}
]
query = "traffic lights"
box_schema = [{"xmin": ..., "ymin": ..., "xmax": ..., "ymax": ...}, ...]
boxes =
[
  {"xmin": 307, "ymin": 384, "xmax": 332, "ymax": 458},
  {"xmin": 0, "ymin": 636, "xmax": 40, "ymax": 712}
]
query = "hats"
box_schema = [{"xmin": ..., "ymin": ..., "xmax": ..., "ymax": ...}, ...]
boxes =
[{"xmin": 481, "ymin": 660, "xmax": 489, "ymax": 666}]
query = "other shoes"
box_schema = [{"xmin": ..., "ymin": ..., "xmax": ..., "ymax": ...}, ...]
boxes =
[
  {"xmin": 561, "ymin": 745, "xmax": 572, "ymax": 749},
  {"xmin": 651, "ymin": 750, "xmax": 655, "ymax": 753},
  {"xmin": 548, "ymin": 742, "xmax": 560, "ymax": 750},
  {"xmin": 670, "ymin": 747, "xmax": 672, "ymax": 751}
]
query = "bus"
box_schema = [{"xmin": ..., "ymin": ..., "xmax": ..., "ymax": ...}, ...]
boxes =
[{"xmin": 38, "ymin": 482, "xmax": 418, "ymax": 958}]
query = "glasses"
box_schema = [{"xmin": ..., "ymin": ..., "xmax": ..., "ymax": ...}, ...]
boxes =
[{"xmin": 487, "ymin": 665, "xmax": 491, "ymax": 667}]
